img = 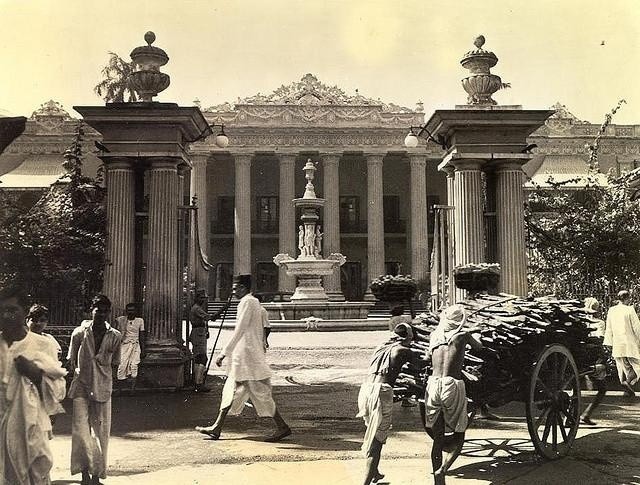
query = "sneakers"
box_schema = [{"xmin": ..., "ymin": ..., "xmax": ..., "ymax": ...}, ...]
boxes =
[
  {"xmin": 195, "ymin": 425, "xmax": 220, "ymax": 440},
  {"xmin": 264, "ymin": 428, "xmax": 291, "ymax": 442}
]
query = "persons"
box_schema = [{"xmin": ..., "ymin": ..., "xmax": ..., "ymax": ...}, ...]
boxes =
[
  {"xmin": 1, "ymin": 287, "xmax": 70, "ymax": 484},
  {"xmin": 424, "ymin": 305, "xmax": 485, "ymax": 484},
  {"xmin": 188, "ymin": 289, "xmax": 232, "ymax": 392},
  {"xmin": 358, "ymin": 323, "xmax": 425, "ymax": 485},
  {"xmin": 583, "ymin": 297, "xmax": 605, "ymax": 392},
  {"xmin": 26, "ymin": 303, "xmax": 62, "ymax": 358},
  {"xmin": 603, "ymin": 290, "xmax": 640, "ymax": 398},
  {"xmin": 388, "ymin": 305, "xmax": 411, "ymax": 332},
  {"xmin": 65, "ymin": 294, "xmax": 120, "ymax": 485},
  {"xmin": 299, "ymin": 225, "xmax": 303, "ymax": 249},
  {"xmin": 115, "ymin": 303, "xmax": 147, "ymax": 394},
  {"xmin": 317, "ymin": 225, "xmax": 324, "ymax": 253},
  {"xmin": 194, "ymin": 272, "xmax": 292, "ymax": 441}
]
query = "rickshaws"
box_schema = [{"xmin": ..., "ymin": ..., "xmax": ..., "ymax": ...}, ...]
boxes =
[{"xmin": 391, "ymin": 342, "xmax": 618, "ymax": 462}]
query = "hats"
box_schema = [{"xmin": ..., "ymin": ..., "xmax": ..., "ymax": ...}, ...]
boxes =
[{"xmin": 233, "ymin": 274, "xmax": 250, "ymax": 284}]
